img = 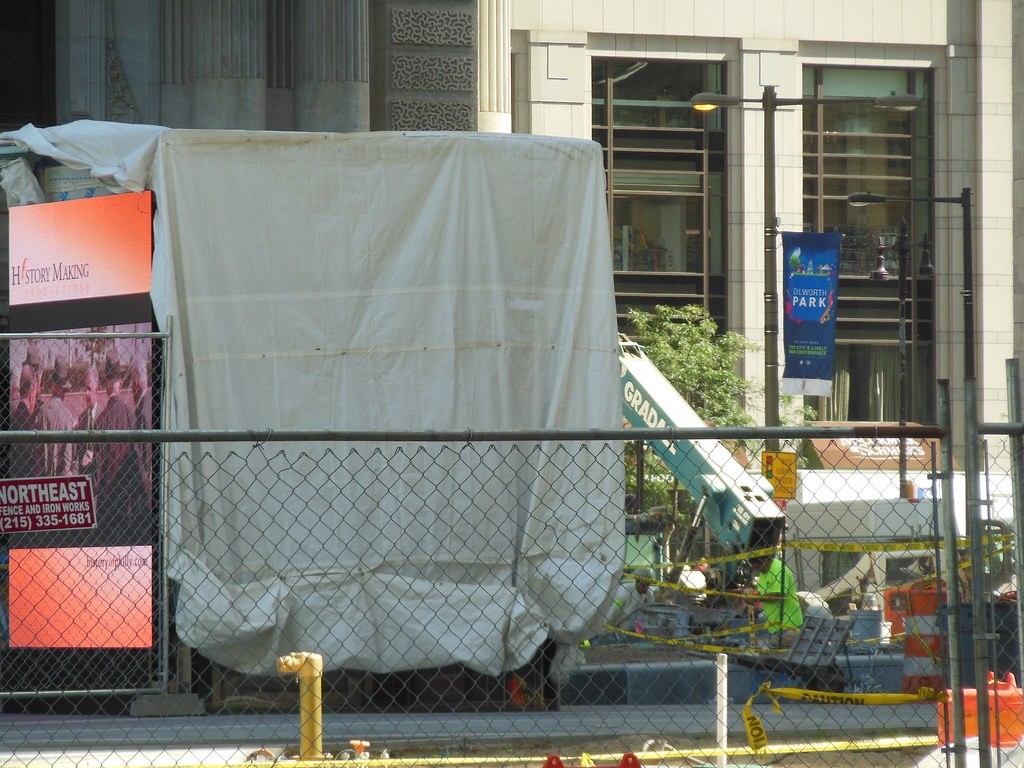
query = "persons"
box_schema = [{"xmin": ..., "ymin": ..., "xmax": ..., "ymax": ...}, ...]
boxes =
[
  {"xmin": 743, "ymin": 557, "xmax": 803, "ymax": 647},
  {"xmin": 9, "ymin": 347, "xmax": 153, "ymax": 546},
  {"xmin": 604, "ymin": 568, "xmax": 654, "ymax": 626}
]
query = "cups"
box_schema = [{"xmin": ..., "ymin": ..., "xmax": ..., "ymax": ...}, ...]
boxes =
[{"xmin": 917, "ymin": 487, "xmax": 928, "ymax": 499}]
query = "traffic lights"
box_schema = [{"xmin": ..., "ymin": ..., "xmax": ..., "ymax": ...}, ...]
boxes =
[{"xmin": 766, "ymin": 454, "xmax": 773, "ymax": 479}]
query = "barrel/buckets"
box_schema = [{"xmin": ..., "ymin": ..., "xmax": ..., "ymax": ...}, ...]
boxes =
[{"xmin": 850, "ymin": 610, "xmax": 882, "ymax": 640}]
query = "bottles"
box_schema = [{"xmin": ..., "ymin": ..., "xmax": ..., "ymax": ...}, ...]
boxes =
[{"xmin": 907, "ymin": 479, "xmax": 916, "ymax": 498}]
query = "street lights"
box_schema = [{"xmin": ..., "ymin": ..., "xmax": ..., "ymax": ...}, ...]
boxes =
[
  {"xmin": 692, "ymin": 85, "xmax": 923, "ymax": 515},
  {"xmin": 848, "ymin": 187, "xmax": 977, "ymax": 384}
]
